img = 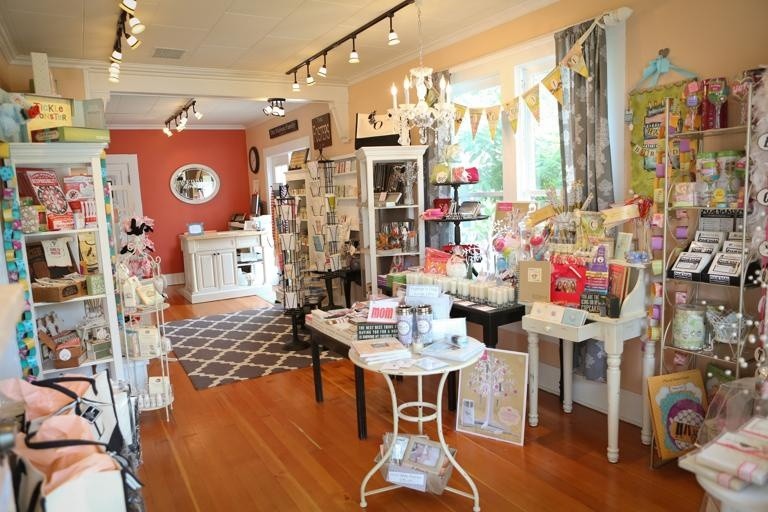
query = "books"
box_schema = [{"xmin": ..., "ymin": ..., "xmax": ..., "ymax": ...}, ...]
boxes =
[
  {"xmin": 304, "ymin": 302, "xmax": 487, "ymax": 371},
  {"xmin": 563, "ymin": 309, "xmax": 586, "ymax": 327},
  {"xmin": 120, "ymin": 284, "xmax": 170, "ymax": 394},
  {"xmin": 284, "ymin": 225, "xmax": 342, "ymax": 309},
  {"xmin": 333, "ymin": 158, "xmax": 356, "ymax": 196},
  {"xmin": 518, "ymin": 261, "xmax": 550, "ymax": 305},
  {"xmin": 581, "ymin": 265, "xmax": 631, "ymax": 312},
  {"xmin": 614, "ymin": 233, "xmax": 633, "ymax": 259},
  {"xmin": 530, "ymin": 302, "xmax": 563, "ymax": 322}
]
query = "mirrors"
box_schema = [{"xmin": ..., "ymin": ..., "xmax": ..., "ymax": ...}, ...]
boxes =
[{"xmin": 170, "ymin": 164, "xmax": 221, "ymax": 205}]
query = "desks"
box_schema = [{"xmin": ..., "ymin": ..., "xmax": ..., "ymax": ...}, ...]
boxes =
[
  {"xmin": 304, "ymin": 306, "xmax": 458, "ymax": 440},
  {"xmin": 522, "ymin": 267, "xmax": 656, "ymax": 463},
  {"xmin": 692, "ymin": 448, "xmax": 768, "ymax": 512},
  {"xmin": 377, "ymin": 283, "xmax": 525, "ymax": 350},
  {"xmin": 348, "ymin": 335, "xmax": 486, "ymax": 512}
]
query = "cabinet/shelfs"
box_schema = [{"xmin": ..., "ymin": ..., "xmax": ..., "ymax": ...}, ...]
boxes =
[
  {"xmin": 428, "ymin": 180, "xmax": 489, "ymax": 244},
  {"xmin": 176, "ymin": 229, "xmax": 266, "ymax": 304},
  {"xmin": 283, "ymin": 167, "xmax": 323, "ymax": 278},
  {"xmin": 1, "ymin": 140, "xmax": 125, "ymax": 386},
  {"xmin": 353, "ymin": 144, "xmax": 426, "ymax": 298},
  {"xmin": 319, "ymin": 153, "xmax": 367, "ymax": 298},
  {"xmin": 649, "ymin": 80, "xmax": 767, "ymax": 471},
  {"xmin": 113, "ymin": 236, "xmax": 175, "ymax": 423}
]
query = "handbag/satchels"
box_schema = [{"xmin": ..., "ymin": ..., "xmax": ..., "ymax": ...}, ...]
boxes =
[
  {"xmin": 24, "ymin": 369, "xmax": 118, "ymax": 451},
  {"xmin": 355, "ymin": 110, "xmax": 401, "ymax": 149},
  {"xmin": 8, "ymin": 426, "xmax": 142, "ymax": 511}
]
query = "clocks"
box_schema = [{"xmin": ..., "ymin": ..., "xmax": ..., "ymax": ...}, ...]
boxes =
[{"xmin": 249, "ymin": 146, "xmax": 259, "ymax": 174}]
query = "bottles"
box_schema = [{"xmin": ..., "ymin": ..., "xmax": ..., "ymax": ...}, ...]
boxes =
[
  {"xmin": 406, "ymin": 271, "xmax": 515, "ymax": 307},
  {"xmin": 717, "ymin": 150, "xmax": 744, "ymax": 208},
  {"xmin": 703, "ymin": 78, "xmax": 727, "ymax": 128},
  {"xmin": 407, "ymin": 230, "xmax": 417, "ymax": 253},
  {"xmin": 682, "ymin": 79, "xmax": 701, "ymax": 130},
  {"xmin": 138, "ymin": 394, "xmax": 163, "ymax": 408},
  {"xmin": 396, "ymin": 305, "xmax": 415, "ymax": 346},
  {"xmin": 694, "ymin": 151, "xmax": 716, "ymax": 206},
  {"xmin": 415, "ymin": 304, "xmax": 432, "ymax": 343}
]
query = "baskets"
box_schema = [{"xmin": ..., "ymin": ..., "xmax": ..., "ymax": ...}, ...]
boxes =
[{"xmin": 705, "ymin": 308, "xmax": 753, "ymax": 343}]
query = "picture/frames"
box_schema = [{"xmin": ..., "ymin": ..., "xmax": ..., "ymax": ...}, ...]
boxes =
[{"xmin": 455, "ymin": 347, "xmax": 530, "ymax": 447}]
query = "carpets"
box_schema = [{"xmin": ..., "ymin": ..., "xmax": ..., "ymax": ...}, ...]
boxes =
[{"xmin": 156, "ymin": 303, "xmax": 345, "ymax": 393}]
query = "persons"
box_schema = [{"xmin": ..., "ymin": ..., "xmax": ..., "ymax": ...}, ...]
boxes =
[{"xmin": 556, "ymin": 281, "xmax": 575, "ymax": 292}]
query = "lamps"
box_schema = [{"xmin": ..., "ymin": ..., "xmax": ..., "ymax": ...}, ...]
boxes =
[
  {"xmin": 284, "ymin": 1, "xmax": 415, "ymax": 92},
  {"xmin": 162, "ymin": 99, "xmax": 204, "ymax": 139},
  {"xmin": 385, "ymin": 3, "xmax": 459, "ymax": 145},
  {"xmin": 107, "ymin": 0, "xmax": 146, "ymax": 84},
  {"xmin": 263, "ymin": 98, "xmax": 286, "ymax": 117}
]
